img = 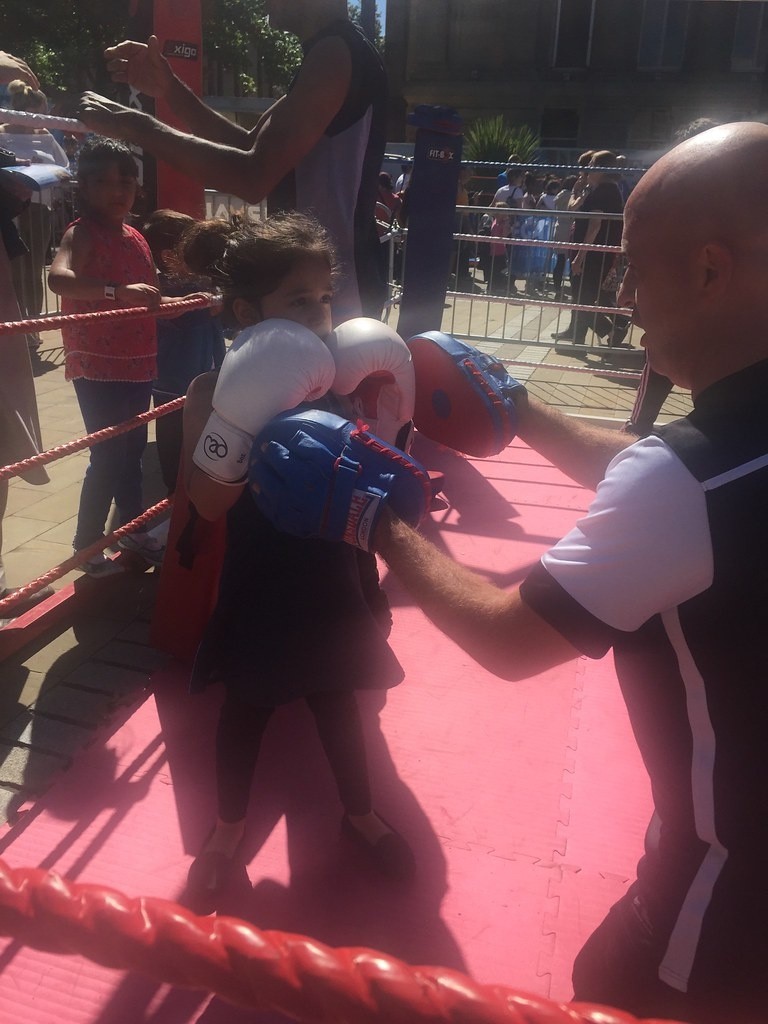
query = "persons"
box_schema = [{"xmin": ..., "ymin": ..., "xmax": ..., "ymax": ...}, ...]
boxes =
[
  {"xmin": 614, "ymin": 115, "xmax": 730, "ymax": 441},
  {"xmin": 244, "ymin": 117, "xmax": 768, "ymax": 1024},
  {"xmin": 77, "ymin": 0, "xmax": 394, "ymax": 646},
  {"xmin": 148, "ymin": 208, "xmax": 417, "ymax": 898},
  {"xmin": 1, "ymin": 50, "xmax": 226, "ymax": 579},
  {"xmin": 375, "ymin": 148, "xmax": 646, "ymax": 349}
]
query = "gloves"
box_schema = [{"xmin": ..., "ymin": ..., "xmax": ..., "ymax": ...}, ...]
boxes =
[
  {"xmin": 192, "ymin": 320, "xmax": 335, "ymax": 485},
  {"xmin": 324, "ymin": 316, "xmax": 416, "ymax": 449}
]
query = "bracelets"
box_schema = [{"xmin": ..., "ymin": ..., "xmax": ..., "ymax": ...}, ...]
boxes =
[{"xmin": 104, "ymin": 280, "xmax": 121, "ymax": 301}]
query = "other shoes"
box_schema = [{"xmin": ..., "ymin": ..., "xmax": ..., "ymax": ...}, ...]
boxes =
[
  {"xmin": 607, "ymin": 327, "xmax": 627, "ymax": 347},
  {"xmin": 187, "ymin": 822, "xmax": 247, "ymax": 898},
  {"xmin": 0, "ymin": 585, "xmax": 55, "ymax": 618},
  {"xmin": 0, "ymin": 617, "xmax": 17, "ymax": 630},
  {"xmin": 551, "ymin": 330, "xmax": 585, "ymax": 344},
  {"xmin": 75, "ymin": 558, "xmax": 125, "ymax": 579},
  {"xmin": 117, "ymin": 535, "xmax": 166, "ymax": 566},
  {"xmin": 342, "ymin": 809, "xmax": 417, "ymax": 882},
  {"xmin": 555, "ymin": 347, "xmax": 587, "ymax": 357}
]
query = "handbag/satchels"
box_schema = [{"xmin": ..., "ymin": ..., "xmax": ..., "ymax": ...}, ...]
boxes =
[
  {"xmin": 601, "ymin": 257, "xmax": 619, "ymax": 291},
  {"xmin": 506, "ymin": 186, "xmax": 519, "ymax": 207}
]
